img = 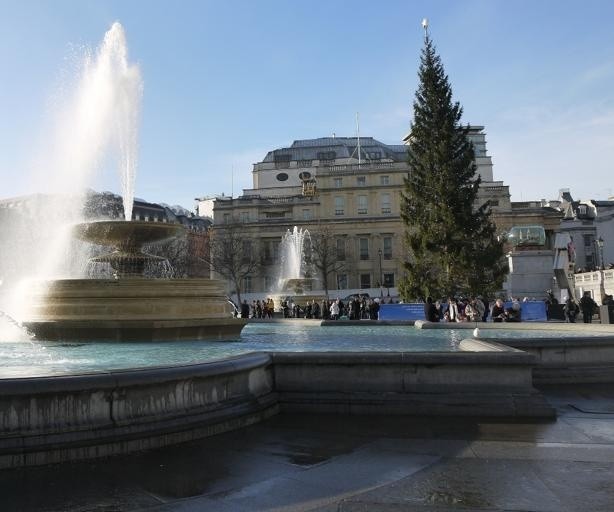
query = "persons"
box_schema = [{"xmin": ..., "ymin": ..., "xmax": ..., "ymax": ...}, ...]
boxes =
[
  {"xmin": 399, "ymin": 298, "xmax": 406, "ymax": 304},
  {"xmin": 379, "ymin": 299, "xmax": 385, "ymax": 304},
  {"xmin": 545, "ymin": 292, "xmax": 613, "ymax": 323},
  {"xmin": 415, "ymin": 292, "xmax": 536, "ymax": 322},
  {"xmin": 242, "ymin": 298, "xmax": 274, "ymax": 319},
  {"xmin": 281, "ymin": 293, "xmax": 380, "ymax": 320},
  {"xmin": 387, "ymin": 298, "xmax": 397, "ymax": 304}
]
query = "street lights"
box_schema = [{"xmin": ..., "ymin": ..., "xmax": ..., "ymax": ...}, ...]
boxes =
[
  {"xmin": 377, "ymin": 248, "xmax": 384, "ymax": 305},
  {"xmin": 596, "ymin": 236, "xmax": 604, "ymax": 269}
]
